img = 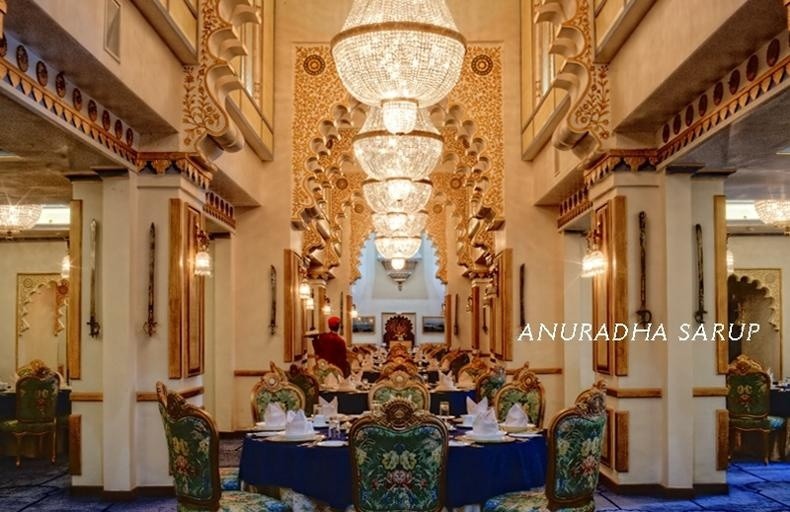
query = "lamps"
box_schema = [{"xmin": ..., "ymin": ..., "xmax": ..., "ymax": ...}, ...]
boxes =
[
  {"xmin": 441, "ymin": 303, "xmax": 446, "ymax": 316},
  {"xmin": 361, "ymin": 174, "xmax": 435, "ymax": 234},
  {"xmin": 329, "ymin": 1, "xmax": 467, "ymax": 134},
  {"xmin": 322, "ymin": 294, "xmax": 336, "ymax": 318},
  {"xmin": 304, "ymin": 294, "xmax": 315, "ymax": 311},
  {"xmin": 60, "ymin": 256, "xmax": 68, "ymax": 285},
  {"xmin": 299, "ymin": 264, "xmax": 312, "ymax": 301},
  {"xmin": 580, "ymin": 226, "xmax": 610, "ymax": 280},
  {"xmin": 482, "ymin": 284, "xmax": 498, "ymax": 309},
  {"xmin": 465, "ymin": 293, "xmax": 475, "ymax": 312},
  {"xmin": 371, "ymin": 237, "xmax": 421, "ymax": 272},
  {"xmin": 349, "ymin": 299, "xmax": 360, "ymax": 321},
  {"xmin": 727, "ymin": 250, "xmax": 734, "ymax": 278},
  {"xmin": 756, "ymin": 196, "xmax": 790, "ymax": 235},
  {"xmin": 192, "ymin": 225, "xmax": 214, "ymax": 277},
  {"xmin": 368, "ymin": 211, "xmax": 430, "ymax": 249},
  {"xmin": 0, "ymin": 203, "xmax": 40, "ymax": 237},
  {"xmin": 349, "ymin": 105, "xmax": 446, "ymax": 202}
]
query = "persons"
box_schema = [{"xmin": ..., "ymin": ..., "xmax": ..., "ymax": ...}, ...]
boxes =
[{"xmin": 318, "ymin": 316, "xmax": 346, "ymax": 369}]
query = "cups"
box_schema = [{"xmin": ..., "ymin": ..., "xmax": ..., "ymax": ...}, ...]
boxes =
[
  {"xmin": 439, "ymin": 401, "xmax": 449, "ymax": 421},
  {"xmin": 461, "ymin": 414, "xmax": 473, "ymax": 426},
  {"xmin": 311, "ymin": 415, "xmax": 325, "ymax": 423}
]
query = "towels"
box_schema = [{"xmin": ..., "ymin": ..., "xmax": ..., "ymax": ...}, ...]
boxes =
[
  {"xmin": 324, "ymin": 373, "xmax": 339, "ymax": 388},
  {"xmin": 467, "ymin": 396, "xmax": 487, "ymax": 414},
  {"xmin": 263, "ymin": 403, "xmax": 285, "ymax": 425},
  {"xmin": 506, "ymin": 404, "xmax": 528, "ymax": 426},
  {"xmin": 351, "ymin": 372, "xmax": 363, "ymax": 385},
  {"xmin": 438, "ymin": 372, "xmax": 452, "ymax": 389},
  {"xmin": 318, "ymin": 396, "xmax": 338, "ymax": 416},
  {"xmin": 475, "ymin": 409, "xmax": 497, "ymax": 433},
  {"xmin": 286, "ymin": 410, "xmax": 308, "ymax": 433}
]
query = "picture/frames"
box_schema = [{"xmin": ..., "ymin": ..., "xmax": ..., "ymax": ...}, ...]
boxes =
[
  {"xmin": 352, "ymin": 316, "xmax": 375, "ymax": 334},
  {"xmin": 102, "ymin": 0, "xmax": 122, "ymax": 66},
  {"xmin": 422, "ymin": 316, "xmax": 446, "ymax": 333}
]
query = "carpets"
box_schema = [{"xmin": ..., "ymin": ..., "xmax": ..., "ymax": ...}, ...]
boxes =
[{"xmin": 1, "ymin": 432, "xmax": 790, "ymax": 511}]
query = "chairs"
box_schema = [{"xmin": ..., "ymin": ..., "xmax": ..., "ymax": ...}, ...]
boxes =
[
  {"xmin": 347, "ymin": 398, "xmax": 452, "ymax": 512},
  {"xmin": 457, "ymin": 360, "xmax": 486, "ymax": 381},
  {"xmin": 376, "ymin": 366, "xmax": 424, "ymax": 392},
  {"xmin": 0, "ymin": 369, "xmax": 61, "ymax": 467},
  {"xmin": 510, "ymin": 359, "xmax": 531, "ymax": 383},
  {"xmin": 476, "ymin": 367, "xmax": 506, "ymax": 402},
  {"xmin": 284, "ymin": 362, "xmax": 319, "ymax": 419},
  {"xmin": 723, "ymin": 356, "xmax": 787, "ymax": 463},
  {"xmin": 153, "ymin": 380, "xmax": 243, "ymax": 492},
  {"xmin": 318, "ymin": 364, "xmax": 343, "ymax": 383},
  {"xmin": 367, "ymin": 370, "xmax": 433, "ymax": 412},
  {"xmin": 481, "ymin": 380, "xmax": 609, "ymax": 512},
  {"xmin": 493, "ymin": 370, "xmax": 545, "ymax": 433},
  {"xmin": 251, "ymin": 372, "xmax": 307, "ymax": 423},
  {"xmin": 162, "ymin": 390, "xmax": 294, "ymax": 512}
]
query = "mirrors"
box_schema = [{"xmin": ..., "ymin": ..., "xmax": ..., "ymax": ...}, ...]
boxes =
[
  {"xmin": 12, "ymin": 271, "xmax": 72, "ymax": 374},
  {"xmin": 725, "ymin": 266, "xmax": 784, "ymax": 383}
]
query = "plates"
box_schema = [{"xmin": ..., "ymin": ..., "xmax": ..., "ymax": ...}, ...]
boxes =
[
  {"xmin": 501, "ymin": 422, "xmax": 536, "ymax": 434},
  {"xmin": 313, "ymin": 422, "xmax": 328, "ymax": 428},
  {"xmin": 314, "ymin": 440, "xmax": 348, "ymax": 447}
]
